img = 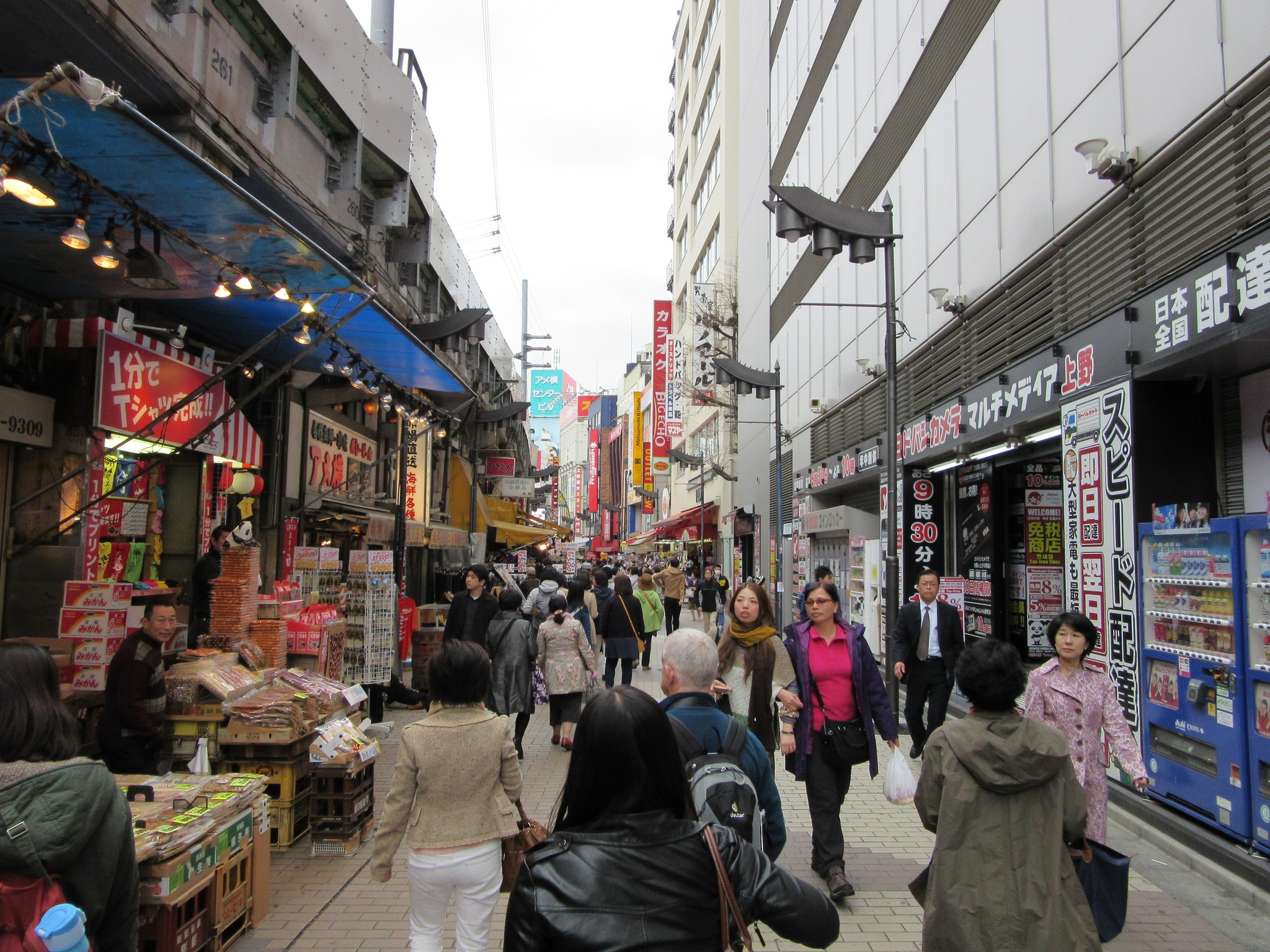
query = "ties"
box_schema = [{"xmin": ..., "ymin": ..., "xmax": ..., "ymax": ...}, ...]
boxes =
[{"xmin": 916, "ymin": 606, "xmax": 930, "ymax": 661}]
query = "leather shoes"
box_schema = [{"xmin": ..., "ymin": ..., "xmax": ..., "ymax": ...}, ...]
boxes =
[
  {"xmin": 550, "ymin": 735, "xmax": 560, "ymax": 744},
  {"xmin": 561, "ymin": 737, "xmax": 573, "ymax": 749}
]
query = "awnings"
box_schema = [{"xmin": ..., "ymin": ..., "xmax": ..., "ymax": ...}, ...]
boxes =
[
  {"xmin": 6, "ymin": 283, "xmax": 481, "ymax": 558},
  {"xmin": 0, "ymin": 57, "xmax": 377, "ymax": 515}
]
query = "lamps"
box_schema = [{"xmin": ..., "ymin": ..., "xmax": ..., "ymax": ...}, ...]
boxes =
[
  {"xmin": 122, "ymin": 217, "xmax": 181, "ymax": 291},
  {"xmin": 408, "ymin": 408, "xmax": 427, "ymax": 425},
  {"xmin": 809, "ymin": 398, "xmax": 821, "ymax": 412},
  {"xmin": 1073, "ymin": 138, "xmax": 1109, "ymax": 175},
  {"xmin": 366, "ymin": 372, "xmax": 383, "ymax": 395},
  {"xmin": 91, "ymin": 216, "xmax": 121, "ymax": 269},
  {"xmin": 214, "ymin": 274, "xmax": 231, "ymax": 298},
  {"xmin": 928, "ymin": 287, "xmax": 949, "ymax": 309},
  {"xmin": 400, "ymin": 405, "xmax": 418, "ymax": 420},
  {"xmin": 122, "ymin": 316, "xmax": 187, "ymax": 349},
  {"xmin": 274, "ymin": 278, "xmax": 289, "ymax": 300},
  {"xmin": 423, "ymin": 419, "xmax": 441, "ymax": 430},
  {"xmin": 339, "ymin": 357, "xmax": 358, "ymax": 376},
  {"xmin": 234, "ymin": 267, "xmax": 253, "ymax": 290},
  {"xmin": 298, "ymin": 293, "xmax": 315, "ymax": 313},
  {"xmin": 418, "ymin": 414, "xmax": 437, "ymax": 425},
  {"xmin": 1, "ymin": 167, "xmax": 58, "ymax": 208},
  {"xmin": 379, "ymin": 392, "xmax": 401, "ymax": 412},
  {"xmin": 350, "ymin": 368, "xmax": 369, "ymax": 389},
  {"xmin": 380, "ymin": 382, "xmax": 394, "ymax": 403},
  {"xmin": 320, "ymin": 349, "xmax": 338, "ymax": 374},
  {"xmin": 316, "ymin": 509, "xmax": 331, "ymax": 522},
  {"xmin": 855, "ymin": 358, "xmax": 871, "ymax": 373},
  {"xmin": 334, "ymin": 507, "xmax": 344, "ymax": 520},
  {"xmin": 437, "ymin": 422, "xmax": 447, "ymax": 437},
  {"xmin": 294, "ymin": 321, "xmax": 312, "ymax": 344},
  {"xmin": 205, "ymin": 354, "xmax": 264, "ymax": 380},
  {"xmin": 60, "ymin": 194, "xmax": 93, "ymax": 249},
  {"xmin": 395, "ymin": 397, "xmax": 413, "ymax": 414}
]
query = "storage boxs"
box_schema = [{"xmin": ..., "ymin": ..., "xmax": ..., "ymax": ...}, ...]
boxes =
[
  {"xmin": 72, "ymin": 637, "xmax": 125, "ymax": 663},
  {"xmin": 133, "ymin": 883, "xmax": 213, "ymax": 952},
  {"xmin": 361, "ymin": 817, "xmax": 377, "ymax": 842},
  {"xmin": 139, "ymin": 832, "xmax": 213, "ymax": 905},
  {"xmin": 62, "ymin": 578, "xmax": 133, "ymax": 609},
  {"xmin": 308, "ymin": 830, "xmax": 361, "ymax": 858},
  {"xmin": 210, "ymin": 805, "xmax": 254, "ymax": 868},
  {"xmin": 266, "ymin": 794, "xmax": 314, "ymax": 852},
  {"xmin": 128, "ymin": 621, "xmax": 188, "ymax": 656},
  {"xmin": 164, "ymin": 696, "xmax": 227, "ymax": 722},
  {"xmin": 211, "ymin": 843, "xmax": 253, "ymax": 937},
  {"xmin": 212, "ymin": 750, "xmax": 314, "ymax": 808},
  {"xmin": 2, "ymin": 635, "xmax": 73, "ymax": 686},
  {"xmin": 409, "ymin": 602, "xmax": 452, "ymax": 690},
  {"xmin": 306, "ymin": 731, "xmax": 379, "ymax": 840},
  {"xmin": 161, "ymin": 720, "xmax": 221, "ymax": 740},
  {"xmin": 74, "ymin": 664, "xmax": 112, "ymax": 691},
  {"xmin": 207, "ymin": 908, "xmax": 253, "ymax": 952},
  {"xmin": 160, "ymin": 739, "xmax": 226, "ymax": 761},
  {"xmin": 58, "ymin": 608, "xmax": 128, "ymax": 638}
]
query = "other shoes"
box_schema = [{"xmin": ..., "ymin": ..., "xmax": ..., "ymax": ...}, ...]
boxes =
[
  {"xmin": 690, "ymin": 618, "xmax": 696, "ymax": 621},
  {"xmin": 826, "ymin": 866, "xmax": 855, "ymax": 899},
  {"xmin": 697, "ymin": 615, "xmax": 701, "ymax": 620}
]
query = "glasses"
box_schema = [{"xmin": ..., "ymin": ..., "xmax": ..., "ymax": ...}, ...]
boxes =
[{"xmin": 804, "ymin": 599, "xmax": 836, "ymax": 606}]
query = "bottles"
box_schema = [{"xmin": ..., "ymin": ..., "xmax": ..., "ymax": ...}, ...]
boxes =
[
  {"xmin": 1150, "ymin": 586, "xmax": 1233, "ymax": 618},
  {"xmin": 1152, "ymin": 617, "xmax": 1234, "ymax": 656},
  {"xmin": 1263, "ymin": 635, "xmax": 1270, "ymax": 663},
  {"xmin": 1260, "ymin": 538, "xmax": 1270, "ymax": 578},
  {"xmin": 1261, "ymin": 588, "xmax": 1270, "ymax": 626},
  {"xmin": 1149, "ymin": 543, "xmax": 1231, "ymax": 581}
]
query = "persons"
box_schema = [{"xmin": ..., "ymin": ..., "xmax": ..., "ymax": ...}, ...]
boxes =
[
  {"xmin": 0, "ymin": 639, "xmax": 139, "ymax": 952},
  {"xmin": 709, "ymin": 582, "xmax": 801, "ymax": 780},
  {"xmin": 1024, "ymin": 612, "xmax": 1150, "ymax": 846},
  {"xmin": 502, "ymin": 682, "xmax": 842, "ymax": 952},
  {"xmin": 656, "ymin": 628, "xmax": 789, "ymax": 864},
  {"xmin": 908, "ymin": 637, "xmax": 1103, "ymax": 952},
  {"xmin": 186, "ymin": 523, "xmax": 233, "ymax": 650},
  {"xmin": 781, "ymin": 580, "xmax": 902, "ymax": 901},
  {"xmin": 407, "ymin": 538, "xmax": 843, "ymax": 759},
  {"xmin": 888, "ymin": 568, "xmax": 963, "ymax": 762},
  {"xmin": 94, "ymin": 595, "xmax": 177, "ymax": 775},
  {"xmin": 370, "ymin": 637, "xmax": 524, "ymax": 951}
]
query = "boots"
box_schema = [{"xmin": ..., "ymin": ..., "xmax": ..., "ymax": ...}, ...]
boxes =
[{"xmin": 512, "ymin": 738, "xmax": 524, "ymax": 759}]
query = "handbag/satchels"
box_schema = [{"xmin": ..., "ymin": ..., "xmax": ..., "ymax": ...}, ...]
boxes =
[
  {"xmin": 586, "ymin": 674, "xmax": 602, "ymax": 704},
  {"xmin": 498, "ymin": 798, "xmax": 553, "ymax": 894},
  {"xmin": 806, "ymin": 666, "xmax": 870, "ymax": 766},
  {"xmin": 637, "ymin": 639, "xmax": 645, "ymax": 652},
  {"xmin": 1067, "ymin": 832, "xmax": 1130, "ymax": 943},
  {"xmin": 532, "ymin": 660, "xmax": 549, "ymax": 705},
  {"xmin": 684, "ymin": 589, "xmax": 694, "ymax": 598},
  {"xmin": 882, "ymin": 745, "xmax": 918, "ymax": 806}
]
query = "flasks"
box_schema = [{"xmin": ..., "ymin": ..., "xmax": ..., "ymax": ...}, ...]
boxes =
[{"xmin": 34, "ymin": 903, "xmax": 90, "ymax": 951}]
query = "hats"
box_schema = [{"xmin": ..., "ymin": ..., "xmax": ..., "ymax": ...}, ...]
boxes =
[{"xmin": 637, "ymin": 573, "xmax": 655, "ymax": 591}]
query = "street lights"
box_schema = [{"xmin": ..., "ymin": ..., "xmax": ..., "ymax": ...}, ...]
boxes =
[
  {"xmin": 469, "ymin": 402, "xmax": 531, "ymax": 544},
  {"xmin": 525, "ymin": 466, "xmax": 561, "ymax": 526},
  {"xmin": 395, "ymin": 307, "xmax": 494, "ymax": 588},
  {"xmin": 600, "ymin": 503, "xmax": 621, "ymax": 553},
  {"xmin": 633, "ymin": 487, "xmax": 660, "ymax": 552},
  {"xmin": 761, "ymin": 185, "xmax": 899, "ymax": 735},
  {"xmin": 710, "ymin": 357, "xmax": 783, "ymax": 643},
  {"xmin": 576, "ymin": 513, "xmax": 592, "ymax": 551},
  {"xmin": 666, "ymin": 448, "xmax": 705, "ymax": 581},
  {"xmin": 561, "ymin": 516, "xmax": 575, "ymax": 543}
]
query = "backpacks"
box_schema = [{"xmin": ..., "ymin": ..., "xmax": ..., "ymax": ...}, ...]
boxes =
[
  {"xmin": 0, "ymin": 870, "xmax": 93, "ymax": 952},
  {"xmin": 663, "ymin": 711, "xmax": 766, "ymax": 854}
]
